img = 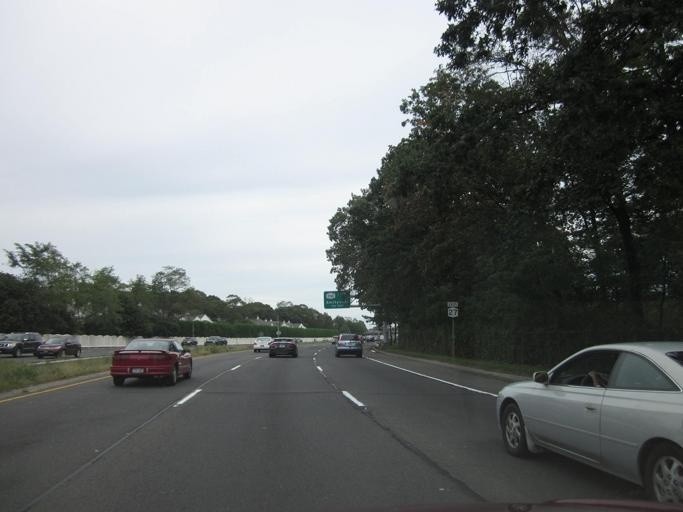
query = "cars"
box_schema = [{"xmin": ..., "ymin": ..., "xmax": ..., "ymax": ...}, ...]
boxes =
[
  {"xmin": 333, "ymin": 334, "xmax": 384, "ymax": 357},
  {"xmin": 496, "ymin": 341, "xmax": 683, "ymax": 505},
  {"xmin": 181, "ymin": 337, "xmax": 198, "ymax": 346},
  {"xmin": 253, "ymin": 336, "xmax": 298, "ymax": 358},
  {"xmin": 111, "ymin": 339, "xmax": 192, "ymax": 386},
  {"xmin": 0, "ymin": 331, "xmax": 81, "ymax": 359},
  {"xmin": 205, "ymin": 336, "xmax": 226, "ymax": 346}
]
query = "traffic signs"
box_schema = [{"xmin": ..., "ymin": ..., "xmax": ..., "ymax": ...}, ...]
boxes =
[
  {"xmin": 447, "ymin": 307, "xmax": 459, "ymax": 318},
  {"xmin": 323, "ymin": 291, "xmax": 350, "ymax": 308}
]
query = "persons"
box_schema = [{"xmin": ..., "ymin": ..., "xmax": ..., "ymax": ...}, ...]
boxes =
[{"xmin": 588, "ymin": 369, "xmax": 608, "ymax": 387}]
